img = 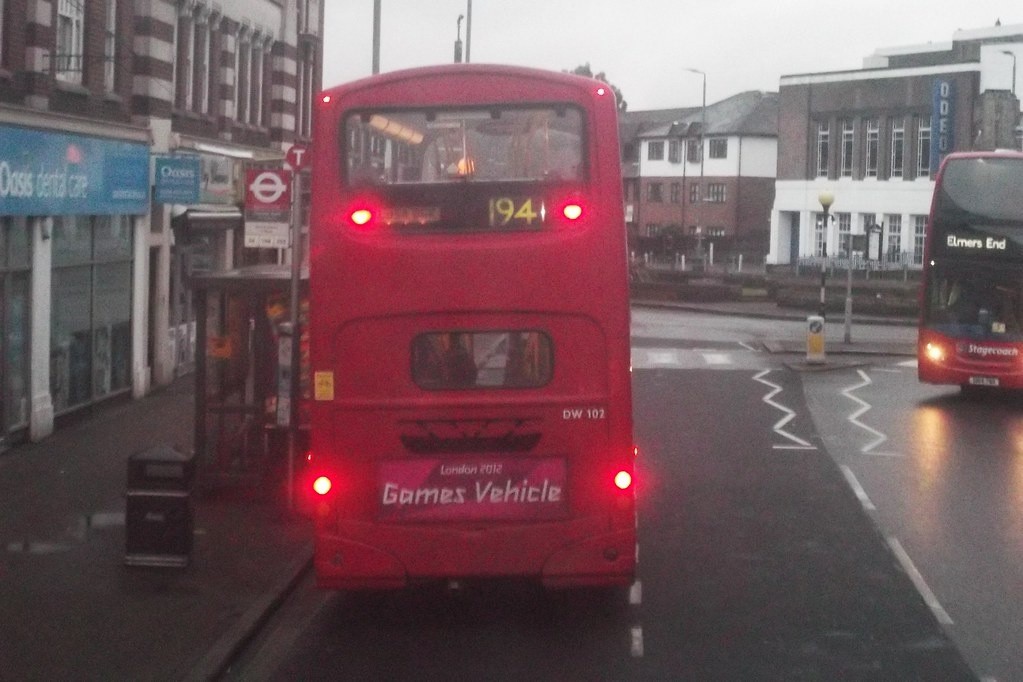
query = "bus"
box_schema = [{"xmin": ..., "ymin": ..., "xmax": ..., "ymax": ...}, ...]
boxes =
[
  {"xmin": 919, "ymin": 148, "xmax": 1023, "ymax": 389},
  {"xmin": 307, "ymin": 64, "xmax": 641, "ymax": 583}
]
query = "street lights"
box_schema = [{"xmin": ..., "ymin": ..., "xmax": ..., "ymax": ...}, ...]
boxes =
[
  {"xmin": 818, "ymin": 191, "xmax": 835, "ymax": 316},
  {"xmin": 686, "ymin": 68, "xmax": 702, "ymax": 279},
  {"xmin": 1003, "ymin": 50, "xmax": 1016, "ymax": 95}
]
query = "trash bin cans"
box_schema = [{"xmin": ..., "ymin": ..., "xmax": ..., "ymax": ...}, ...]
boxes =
[{"xmin": 121, "ymin": 442, "xmax": 194, "ymax": 569}]
